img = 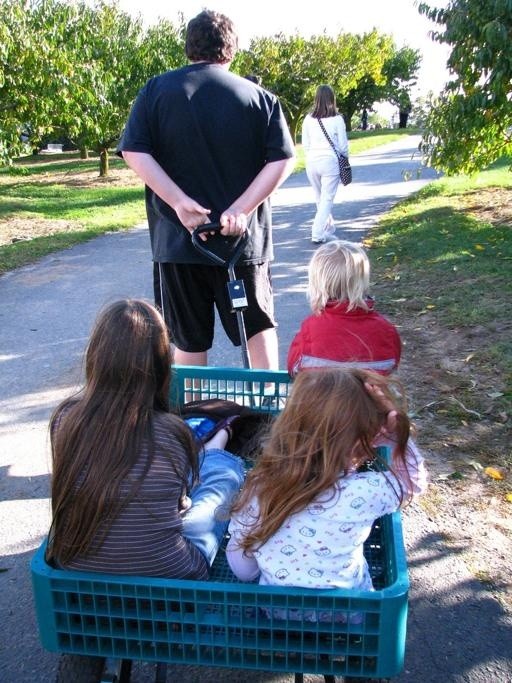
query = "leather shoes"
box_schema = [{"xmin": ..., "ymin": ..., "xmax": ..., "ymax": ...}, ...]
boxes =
[{"xmin": 202, "ymin": 415, "xmax": 240, "ymax": 448}]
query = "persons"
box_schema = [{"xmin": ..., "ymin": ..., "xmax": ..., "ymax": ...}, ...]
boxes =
[
  {"xmin": 47, "ymin": 295, "xmax": 250, "ymax": 581},
  {"xmin": 114, "ymin": 9, "xmax": 300, "ymax": 402},
  {"xmin": 244, "ymin": 74, "xmax": 263, "ymax": 86},
  {"xmin": 301, "ymin": 83, "xmax": 349, "ymax": 244},
  {"xmin": 287, "ymin": 238, "xmax": 403, "ymax": 378},
  {"xmin": 361, "ymin": 109, "xmax": 369, "ymax": 129},
  {"xmin": 398, "ymin": 92, "xmax": 412, "ymax": 128},
  {"xmin": 226, "ymin": 365, "xmax": 431, "ymax": 623}
]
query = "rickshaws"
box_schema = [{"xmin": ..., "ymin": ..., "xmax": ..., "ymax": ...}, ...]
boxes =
[{"xmin": 28, "ymin": 222, "xmax": 411, "ymax": 683}]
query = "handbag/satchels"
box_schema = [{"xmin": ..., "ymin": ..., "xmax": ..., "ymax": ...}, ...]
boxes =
[{"xmin": 339, "ymin": 157, "xmax": 352, "ymax": 185}]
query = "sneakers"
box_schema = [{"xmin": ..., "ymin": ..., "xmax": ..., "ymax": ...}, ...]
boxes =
[{"xmin": 312, "ymin": 237, "xmax": 327, "ymax": 242}]
